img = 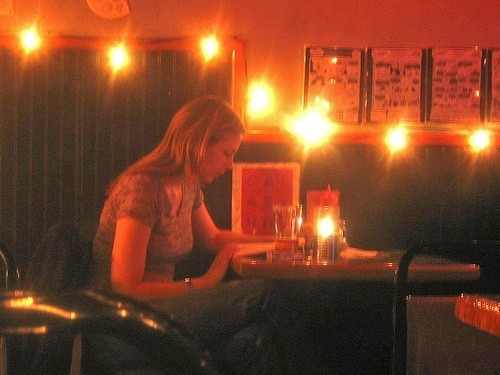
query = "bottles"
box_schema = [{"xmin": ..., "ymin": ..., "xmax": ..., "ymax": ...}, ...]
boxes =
[
  {"xmin": 339, "ymin": 219, "xmax": 346, "ymax": 250},
  {"xmin": 296, "ymin": 204, "xmax": 304, "ymax": 236},
  {"xmin": 316, "ymin": 206, "xmax": 334, "ymax": 264}
]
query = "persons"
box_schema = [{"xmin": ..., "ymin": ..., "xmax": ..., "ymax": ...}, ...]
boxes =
[{"xmin": 83, "ymin": 96, "xmax": 297, "ymax": 375}]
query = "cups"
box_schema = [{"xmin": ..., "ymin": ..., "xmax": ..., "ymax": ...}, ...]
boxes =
[{"xmin": 272, "ymin": 205, "xmax": 300, "ymax": 262}]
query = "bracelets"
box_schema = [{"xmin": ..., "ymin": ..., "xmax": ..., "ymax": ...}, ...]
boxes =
[{"xmin": 184, "ymin": 277, "xmax": 194, "ymax": 291}]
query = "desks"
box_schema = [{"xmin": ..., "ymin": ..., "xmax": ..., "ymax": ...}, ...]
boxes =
[{"xmin": 228, "ymin": 249, "xmax": 481, "ymax": 285}]
